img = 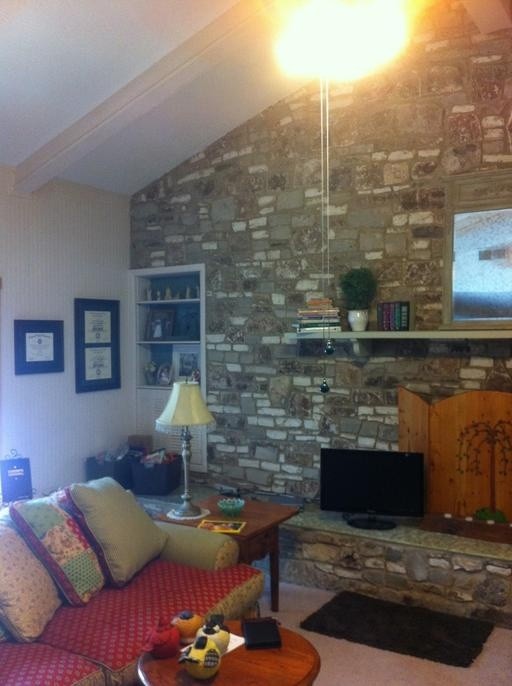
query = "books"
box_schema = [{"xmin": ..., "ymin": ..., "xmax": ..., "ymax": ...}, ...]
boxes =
[
  {"xmin": 376, "ymin": 301, "xmax": 416, "ymax": 331},
  {"xmin": 296, "ymin": 297, "xmax": 341, "ymax": 333}
]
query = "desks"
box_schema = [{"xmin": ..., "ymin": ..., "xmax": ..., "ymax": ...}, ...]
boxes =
[{"xmin": 154, "ymin": 494, "xmax": 300, "ymax": 613}]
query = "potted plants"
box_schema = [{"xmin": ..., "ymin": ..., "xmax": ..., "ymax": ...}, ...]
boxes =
[{"xmin": 341, "ymin": 266, "xmax": 380, "ymax": 331}]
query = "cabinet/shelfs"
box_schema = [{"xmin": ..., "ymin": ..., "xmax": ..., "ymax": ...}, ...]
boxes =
[
  {"xmin": 132, "ymin": 262, "xmax": 206, "ymax": 472},
  {"xmin": 289, "ymin": 325, "xmax": 512, "ymax": 363}
]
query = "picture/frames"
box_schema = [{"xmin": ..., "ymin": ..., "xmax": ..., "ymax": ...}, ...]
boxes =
[
  {"xmin": 442, "ymin": 202, "xmax": 512, "ymax": 332},
  {"xmin": 74, "ymin": 298, "xmax": 123, "ymax": 395},
  {"xmin": 14, "ymin": 318, "xmax": 66, "ymax": 375}
]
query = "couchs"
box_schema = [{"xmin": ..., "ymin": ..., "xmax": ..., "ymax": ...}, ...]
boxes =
[{"xmin": 0, "ymin": 475, "xmax": 266, "ymax": 686}]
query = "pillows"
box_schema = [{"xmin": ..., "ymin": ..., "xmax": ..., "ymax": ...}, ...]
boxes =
[
  {"xmin": 63, "ymin": 476, "xmax": 170, "ymax": 587},
  {"xmin": 0, "ymin": 507, "xmax": 66, "ymax": 642},
  {"xmin": 9, "ymin": 488, "xmax": 109, "ymax": 608}
]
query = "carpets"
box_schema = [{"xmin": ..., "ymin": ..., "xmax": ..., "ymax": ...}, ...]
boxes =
[{"xmin": 300, "ymin": 591, "xmax": 495, "ymax": 670}]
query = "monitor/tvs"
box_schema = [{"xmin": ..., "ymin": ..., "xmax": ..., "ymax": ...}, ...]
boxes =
[{"xmin": 319, "ymin": 447, "xmax": 427, "ymax": 531}]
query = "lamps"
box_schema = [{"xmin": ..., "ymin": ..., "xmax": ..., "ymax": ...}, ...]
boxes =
[{"xmin": 155, "ymin": 379, "xmax": 216, "ymax": 519}]
